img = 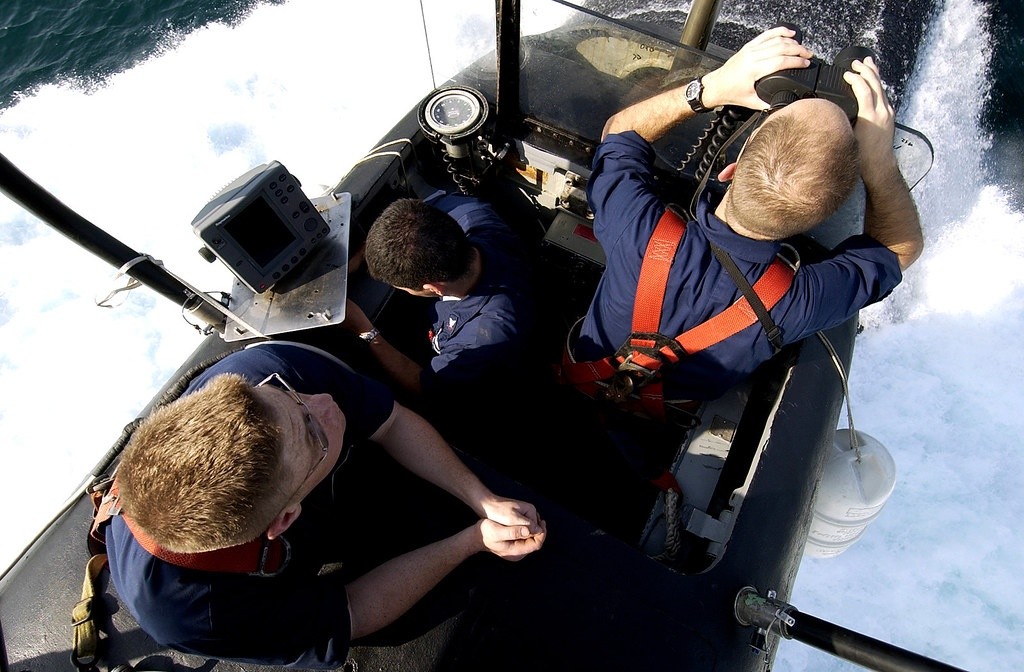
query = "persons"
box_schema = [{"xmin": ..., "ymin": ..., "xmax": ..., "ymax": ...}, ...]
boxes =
[
  {"xmin": 347, "ymin": 191, "xmax": 528, "ymax": 430},
  {"xmin": 104, "ymin": 346, "xmax": 549, "ymax": 668},
  {"xmin": 567, "ymin": 15, "xmax": 920, "ymax": 505}
]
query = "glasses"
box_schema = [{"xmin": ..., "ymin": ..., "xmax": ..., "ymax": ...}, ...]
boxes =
[{"xmin": 254, "ymin": 373, "xmax": 329, "ymax": 535}]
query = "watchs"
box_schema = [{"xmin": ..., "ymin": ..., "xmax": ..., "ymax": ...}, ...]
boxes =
[{"xmin": 686, "ymin": 77, "xmax": 712, "ymax": 114}]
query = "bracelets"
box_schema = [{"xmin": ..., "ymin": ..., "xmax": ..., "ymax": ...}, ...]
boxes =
[{"xmin": 359, "ymin": 328, "xmax": 380, "ymax": 344}]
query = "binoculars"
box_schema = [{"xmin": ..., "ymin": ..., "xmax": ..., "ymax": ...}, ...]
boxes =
[{"xmin": 754, "ymin": 21, "xmax": 875, "ymax": 126}]
